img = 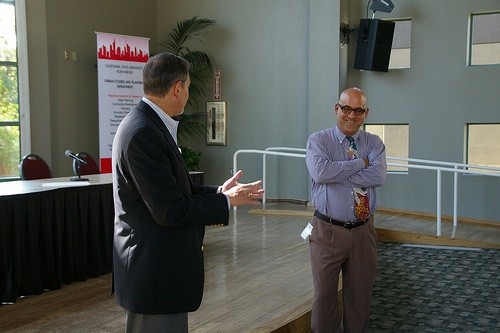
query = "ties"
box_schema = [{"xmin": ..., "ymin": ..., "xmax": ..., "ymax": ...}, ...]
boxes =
[{"xmin": 344, "ymin": 135, "xmax": 370, "ymax": 221}]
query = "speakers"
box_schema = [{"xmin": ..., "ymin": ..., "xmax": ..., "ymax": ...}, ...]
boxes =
[{"xmin": 352, "ymin": 18, "xmax": 396, "ymax": 73}]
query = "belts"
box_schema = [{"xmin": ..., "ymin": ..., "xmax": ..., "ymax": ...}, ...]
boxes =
[{"xmin": 315, "ymin": 211, "xmax": 369, "ymax": 229}]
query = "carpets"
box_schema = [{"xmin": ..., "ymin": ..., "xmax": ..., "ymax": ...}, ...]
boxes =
[{"xmin": 368, "ymin": 241, "xmax": 500, "ymax": 333}]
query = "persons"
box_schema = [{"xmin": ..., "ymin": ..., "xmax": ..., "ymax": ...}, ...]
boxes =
[
  {"xmin": 112, "ymin": 52, "xmax": 266, "ymax": 333},
  {"xmin": 306, "ymin": 87, "xmax": 387, "ymax": 333}
]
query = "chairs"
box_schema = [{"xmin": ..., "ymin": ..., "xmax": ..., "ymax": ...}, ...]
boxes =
[
  {"xmin": 20, "ymin": 153, "xmax": 52, "ymax": 181},
  {"xmin": 72, "ymin": 152, "xmax": 100, "ymax": 176}
]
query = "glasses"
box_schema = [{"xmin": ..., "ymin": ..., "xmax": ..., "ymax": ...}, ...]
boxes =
[{"xmin": 337, "ymin": 103, "xmax": 366, "ymax": 117}]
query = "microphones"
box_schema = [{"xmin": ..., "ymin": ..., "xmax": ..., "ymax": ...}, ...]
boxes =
[{"xmin": 65, "ymin": 149, "xmax": 87, "ymax": 164}]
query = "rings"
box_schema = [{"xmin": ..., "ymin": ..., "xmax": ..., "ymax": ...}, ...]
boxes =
[{"xmin": 248, "ymin": 193, "xmax": 252, "ymax": 198}]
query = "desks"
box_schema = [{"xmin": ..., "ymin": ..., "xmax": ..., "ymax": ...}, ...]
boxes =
[{"xmin": 0, "ymin": 172, "xmax": 204, "ymax": 306}]
